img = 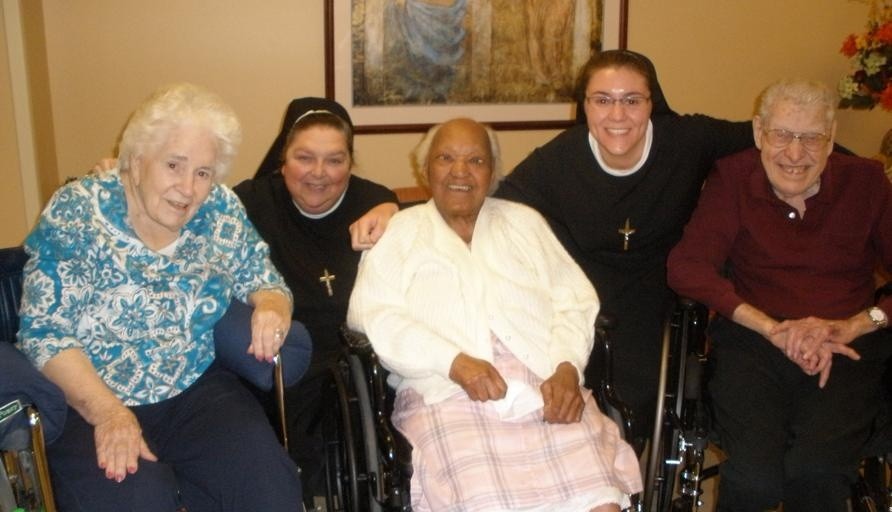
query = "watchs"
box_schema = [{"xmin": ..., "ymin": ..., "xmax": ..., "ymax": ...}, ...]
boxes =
[{"xmin": 863, "ymin": 303, "xmax": 887, "ymax": 331}]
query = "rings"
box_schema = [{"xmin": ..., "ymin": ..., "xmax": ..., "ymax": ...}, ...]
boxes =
[{"xmin": 276, "ymin": 328, "xmax": 286, "ymax": 341}]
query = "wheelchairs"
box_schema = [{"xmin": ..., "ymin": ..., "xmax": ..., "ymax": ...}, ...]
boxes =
[
  {"xmin": 0, "ymin": 244, "xmax": 286, "ymax": 511},
  {"xmin": 312, "ymin": 325, "xmax": 649, "ymax": 512},
  {"xmin": 638, "ymin": 294, "xmax": 892, "ymax": 510}
]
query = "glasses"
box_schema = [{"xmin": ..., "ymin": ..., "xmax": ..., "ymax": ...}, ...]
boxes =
[
  {"xmin": 763, "ymin": 128, "xmax": 832, "ymax": 152},
  {"xmin": 584, "ymin": 87, "xmax": 652, "ymax": 111}
]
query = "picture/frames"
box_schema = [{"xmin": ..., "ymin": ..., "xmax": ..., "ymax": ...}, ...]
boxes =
[{"xmin": 323, "ymin": 0, "xmax": 628, "ymax": 135}]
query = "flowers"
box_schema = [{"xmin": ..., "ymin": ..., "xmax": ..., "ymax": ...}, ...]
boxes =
[{"xmin": 835, "ymin": 2, "xmax": 891, "ymax": 110}]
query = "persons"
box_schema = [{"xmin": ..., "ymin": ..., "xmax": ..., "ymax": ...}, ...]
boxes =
[
  {"xmin": 663, "ymin": 68, "xmax": 892, "ymax": 509},
  {"xmin": 342, "ymin": 45, "xmax": 892, "ymax": 511},
  {"xmin": 11, "ymin": 75, "xmax": 305, "ymax": 510},
  {"xmin": 86, "ymin": 92, "xmax": 400, "ymax": 511},
  {"xmin": 339, "ymin": 111, "xmax": 647, "ymax": 512}
]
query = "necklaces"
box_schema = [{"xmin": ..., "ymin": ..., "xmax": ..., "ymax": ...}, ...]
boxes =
[
  {"xmin": 596, "ymin": 156, "xmax": 655, "ymax": 255},
  {"xmin": 305, "ymin": 231, "xmax": 340, "ymax": 300}
]
